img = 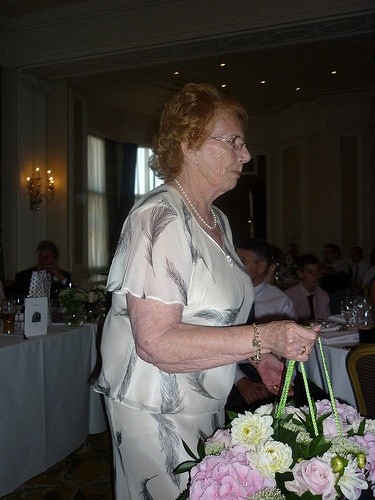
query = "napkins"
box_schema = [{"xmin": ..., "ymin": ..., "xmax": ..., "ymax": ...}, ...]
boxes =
[{"xmin": 321, "ymin": 331, "xmax": 359, "ymax": 345}]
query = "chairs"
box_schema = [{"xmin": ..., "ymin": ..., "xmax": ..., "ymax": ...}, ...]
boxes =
[{"xmin": 346, "ymin": 343, "xmax": 375, "ymax": 419}]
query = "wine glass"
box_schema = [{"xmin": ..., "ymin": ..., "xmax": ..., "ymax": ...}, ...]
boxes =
[{"xmin": 12, "ymin": 295, "xmax": 24, "ymax": 323}]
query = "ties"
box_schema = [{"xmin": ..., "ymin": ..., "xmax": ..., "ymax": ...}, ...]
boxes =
[
  {"xmin": 307, "ymin": 295, "xmax": 315, "ymax": 320},
  {"xmin": 246, "ymin": 302, "xmax": 256, "ymax": 325}
]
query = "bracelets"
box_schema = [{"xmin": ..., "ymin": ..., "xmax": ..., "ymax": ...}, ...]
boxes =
[{"xmin": 250, "ymin": 322, "xmax": 261, "ymax": 365}]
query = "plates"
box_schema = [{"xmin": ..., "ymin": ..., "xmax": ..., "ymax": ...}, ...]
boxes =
[{"xmin": 304, "ymin": 320, "xmax": 342, "ymax": 332}]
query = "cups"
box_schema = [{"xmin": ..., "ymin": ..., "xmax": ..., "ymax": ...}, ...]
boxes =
[
  {"xmin": 14, "ymin": 313, "xmax": 24, "ymax": 321},
  {"xmin": 2, "ymin": 299, "xmax": 16, "ymax": 334},
  {"xmin": 339, "ymin": 296, "xmax": 374, "ymax": 330},
  {"xmin": 14, "ymin": 323, "xmax": 24, "ymax": 334}
]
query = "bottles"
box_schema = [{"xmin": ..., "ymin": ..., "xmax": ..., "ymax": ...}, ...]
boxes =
[{"xmin": 71, "ymin": 314, "xmax": 79, "ymax": 328}]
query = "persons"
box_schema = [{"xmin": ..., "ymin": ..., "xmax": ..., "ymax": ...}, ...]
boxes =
[
  {"xmin": 226, "ymin": 238, "xmax": 330, "ymax": 430},
  {"xmin": 6, "ymin": 240, "xmax": 73, "ymax": 301},
  {"xmin": 349, "ymin": 246, "xmax": 371, "ymax": 283},
  {"xmin": 90, "ymin": 83, "xmax": 321, "ymax": 500},
  {"xmin": 284, "ymin": 254, "xmax": 331, "ymax": 322},
  {"xmin": 284, "ymin": 243, "xmax": 299, "ymax": 266},
  {"xmin": 320, "ymin": 243, "xmax": 351, "ymax": 294},
  {"xmin": 356, "ymin": 248, "xmax": 375, "ymax": 296}
]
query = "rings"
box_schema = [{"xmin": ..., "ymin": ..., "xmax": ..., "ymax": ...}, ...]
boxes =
[
  {"xmin": 301, "ymin": 346, "xmax": 306, "ymax": 353},
  {"xmin": 274, "ymin": 385, "xmax": 278, "ymax": 388}
]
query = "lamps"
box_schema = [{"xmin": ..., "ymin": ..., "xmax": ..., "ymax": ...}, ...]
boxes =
[{"xmin": 26, "ymin": 167, "xmax": 55, "ymax": 214}]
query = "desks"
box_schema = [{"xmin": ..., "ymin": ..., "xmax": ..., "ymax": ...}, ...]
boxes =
[
  {"xmin": 0, "ymin": 322, "xmax": 107, "ymax": 497},
  {"xmin": 297, "ymin": 343, "xmax": 355, "ymax": 408}
]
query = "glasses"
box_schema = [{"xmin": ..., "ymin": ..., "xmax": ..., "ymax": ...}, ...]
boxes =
[{"xmin": 207, "ymin": 134, "xmax": 246, "ymax": 151}]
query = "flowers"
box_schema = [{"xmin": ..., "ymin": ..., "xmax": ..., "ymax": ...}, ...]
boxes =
[
  {"xmin": 171, "ymin": 398, "xmax": 375, "ymax": 500},
  {"xmin": 58, "ymin": 285, "xmax": 105, "ymax": 310}
]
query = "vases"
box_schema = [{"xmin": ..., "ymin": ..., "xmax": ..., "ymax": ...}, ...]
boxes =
[{"xmin": 62, "ymin": 307, "xmax": 84, "ymax": 328}]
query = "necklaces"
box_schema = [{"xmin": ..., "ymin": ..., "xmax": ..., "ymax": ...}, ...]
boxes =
[{"xmin": 175, "ymin": 178, "xmax": 217, "ymax": 229}]
